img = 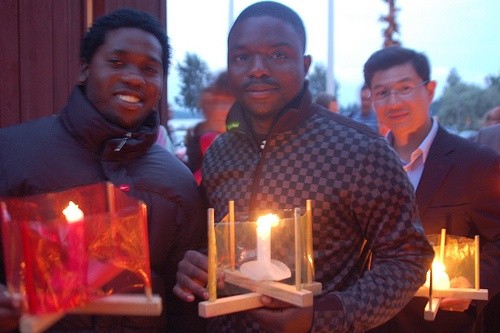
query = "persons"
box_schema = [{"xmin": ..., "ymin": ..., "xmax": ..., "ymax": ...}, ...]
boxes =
[
  {"xmin": 360, "ymin": 45, "xmax": 500, "ymax": 333},
  {"xmin": 0, "ymin": 7, "xmax": 207, "ymax": 332},
  {"xmin": 170, "ymin": 1, "xmax": 434, "ymax": 333},
  {"xmin": 185, "ymin": 68, "xmax": 500, "ymax": 184}
]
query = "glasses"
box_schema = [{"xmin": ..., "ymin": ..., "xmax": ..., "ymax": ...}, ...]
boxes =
[{"xmin": 366, "ymin": 81, "xmax": 430, "ymax": 100}]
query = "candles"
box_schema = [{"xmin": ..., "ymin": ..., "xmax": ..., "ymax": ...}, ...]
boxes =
[
  {"xmin": 256, "ymin": 213, "xmax": 280, "ymax": 272},
  {"xmin": 65, "ymin": 202, "xmax": 86, "ymax": 280},
  {"xmin": 431, "ymin": 257, "xmax": 450, "ymax": 292}
]
